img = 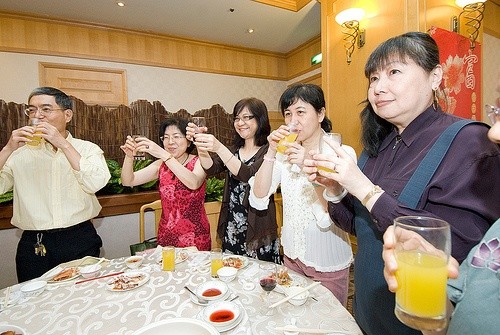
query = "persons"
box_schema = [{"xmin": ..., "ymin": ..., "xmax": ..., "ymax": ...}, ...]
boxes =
[
  {"xmin": 302, "ymin": 33, "xmax": 500, "ymax": 335},
  {"xmin": 186, "ymin": 97, "xmax": 282, "ymax": 264},
  {"xmin": 248, "ymin": 85, "xmax": 354, "ymax": 311},
  {"xmin": 0, "ymin": 87, "xmax": 113, "ymax": 286},
  {"xmin": 381, "ymin": 100, "xmax": 500, "ymax": 335},
  {"xmin": 122, "ymin": 117, "xmax": 212, "ymax": 251}
]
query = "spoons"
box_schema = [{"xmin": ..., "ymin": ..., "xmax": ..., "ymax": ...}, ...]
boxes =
[{"xmin": 184, "ymin": 285, "xmax": 208, "ymax": 305}]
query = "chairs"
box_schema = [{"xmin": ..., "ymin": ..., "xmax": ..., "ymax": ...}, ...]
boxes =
[{"xmin": 139, "ymin": 199, "xmax": 162, "ymax": 243}]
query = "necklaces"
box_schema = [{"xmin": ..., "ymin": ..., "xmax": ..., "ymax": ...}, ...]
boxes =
[{"xmin": 181, "ymin": 154, "xmax": 190, "ymax": 167}]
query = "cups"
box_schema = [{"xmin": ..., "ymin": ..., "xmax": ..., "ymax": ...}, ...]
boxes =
[
  {"xmin": 320, "ymin": 132, "xmax": 342, "ymax": 176},
  {"xmin": 190, "ymin": 117, "xmax": 206, "ymax": 141},
  {"xmin": 161, "ymin": 246, "xmax": 175, "ymax": 271},
  {"xmin": 276, "ymin": 124, "xmax": 300, "ymax": 155},
  {"xmin": 132, "ymin": 136, "xmax": 146, "ymax": 162},
  {"xmin": 394, "ymin": 217, "xmax": 451, "ymax": 332},
  {"xmin": 25, "ymin": 118, "xmax": 44, "ymax": 147},
  {"xmin": 210, "ymin": 249, "xmax": 224, "ymax": 278}
]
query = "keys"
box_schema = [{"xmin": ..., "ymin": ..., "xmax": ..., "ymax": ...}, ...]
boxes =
[{"xmin": 33, "ymin": 241, "xmax": 46, "ymax": 256}]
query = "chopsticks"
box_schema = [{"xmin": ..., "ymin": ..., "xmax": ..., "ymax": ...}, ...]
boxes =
[
  {"xmin": 75, "ymin": 271, "xmax": 123, "ymax": 285},
  {"xmin": 4, "ymin": 287, "xmax": 10, "ymax": 307},
  {"xmin": 267, "ymin": 280, "xmax": 321, "ymax": 309}
]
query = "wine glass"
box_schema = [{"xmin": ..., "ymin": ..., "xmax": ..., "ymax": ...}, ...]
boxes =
[{"xmin": 259, "ymin": 261, "xmax": 277, "ymax": 317}]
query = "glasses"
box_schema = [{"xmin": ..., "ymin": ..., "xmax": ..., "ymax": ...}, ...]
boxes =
[
  {"xmin": 485, "ymin": 104, "xmax": 500, "ymax": 125},
  {"xmin": 232, "ymin": 114, "xmax": 255, "ymax": 123},
  {"xmin": 24, "ymin": 106, "xmax": 65, "ymax": 117},
  {"xmin": 160, "ymin": 134, "xmax": 186, "ymax": 142}
]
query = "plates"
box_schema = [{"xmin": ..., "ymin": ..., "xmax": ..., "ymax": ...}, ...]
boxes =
[
  {"xmin": 223, "ymin": 254, "xmax": 250, "ymax": 270},
  {"xmin": 197, "ymin": 308, "xmax": 242, "ymax": 333},
  {"xmin": 106, "ymin": 269, "xmax": 150, "ymax": 291},
  {"xmin": 275, "ymin": 272, "xmax": 307, "ymax": 293},
  {"xmin": 47, "ymin": 271, "xmax": 80, "ymax": 283},
  {"xmin": 190, "ymin": 287, "xmax": 233, "ymax": 305},
  {"xmin": 155, "ymin": 250, "xmax": 189, "ymax": 265}
]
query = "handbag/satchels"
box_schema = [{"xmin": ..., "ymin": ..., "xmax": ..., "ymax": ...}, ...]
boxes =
[{"xmin": 129, "ymin": 236, "xmax": 159, "ymax": 256}]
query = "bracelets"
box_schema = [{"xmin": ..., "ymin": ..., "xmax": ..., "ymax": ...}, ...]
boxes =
[
  {"xmin": 361, "ymin": 184, "xmax": 384, "ymax": 205},
  {"xmin": 223, "ymin": 154, "xmax": 234, "ymax": 166},
  {"xmin": 323, "ymin": 188, "xmax": 348, "ymax": 202},
  {"xmin": 262, "ymin": 154, "xmax": 276, "ymax": 161},
  {"xmin": 164, "ymin": 154, "xmax": 173, "ymax": 163}
]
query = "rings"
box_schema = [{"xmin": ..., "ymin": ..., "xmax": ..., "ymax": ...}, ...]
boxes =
[
  {"xmin": 334, "ymin": 164, "xmax": 337, "ymax": 171},
  {"xmin": 146, "ymin": 145, "xmax": 149, "ymax": 149}
]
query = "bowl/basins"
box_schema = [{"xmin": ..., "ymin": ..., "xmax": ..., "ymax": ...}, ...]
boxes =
[
  {"xmin": 196, "ymin": 281, "xmax": 228, "ymax": 300},
  {"xmin": 125, "ymin": 256, "xmax": 143, "ymax": 269},
  {"xmin": 286, "ymin": 287, "xmax": 310, "ymax": 306},
  {"xmin": 217, "ymin": 267, "xmax": 237, "ymax": 282},
  {"xmin": 0, "ymin": 324, "xmax": 25, "ymax": 335},
  {"xmin": 79, "ymin": 264, "xmax": 102, "ymax": 278},
  {"xmin": 20, "ymin": 280, "xmax": 47, "ymax": 298},
  {"xmin": 204, "ymin": 302, "xmax": 240, "ymax": 326}
]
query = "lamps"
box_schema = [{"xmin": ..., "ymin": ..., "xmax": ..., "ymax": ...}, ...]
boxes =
[
  {"xmin": 454, "ymin": 0, "xmax": 487, "ymax": 51},
  {"xmin": 335, "ymin": 8, "xmax": 366, "ymax": 65}
]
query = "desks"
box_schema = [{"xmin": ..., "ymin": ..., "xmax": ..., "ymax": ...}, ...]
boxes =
[{"xmin": 0, "ymin": 246, "xmax": 364, "ymax": 335}]
